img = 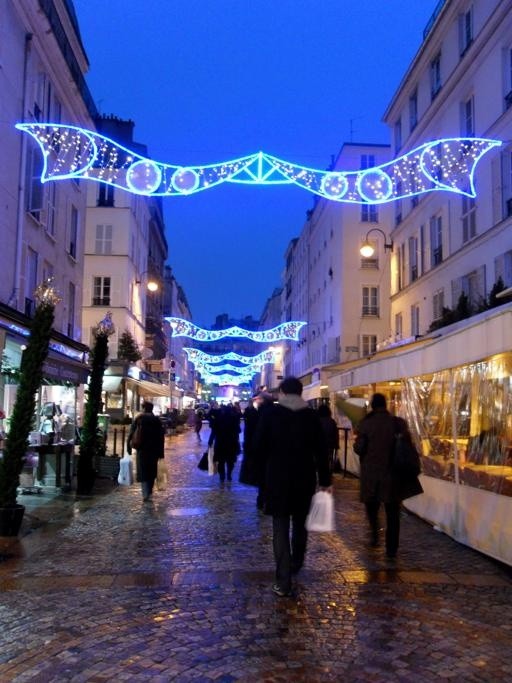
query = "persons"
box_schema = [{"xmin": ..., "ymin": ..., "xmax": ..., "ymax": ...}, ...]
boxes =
[
  {"xmin": 126, "ymin": 399, "xmax": 164, "ymax": 500},
  {"xmin": 251, "ymin": 375, "xmax": 335, "ymax": 596},
  {"xmin": 194, "ymin": 398, "xmax": 340, "ymax": 481},
  {"xmin": 352, "ymin": 391, "xmax": 413, "ymax": 557}
]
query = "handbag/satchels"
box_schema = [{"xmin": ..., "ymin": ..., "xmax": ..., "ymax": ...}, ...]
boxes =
[
  {"xmin": 129, "ymin": 413, "xmax": 141, "ymax": 449},
  {"xmin": 197, "ymin": 444, "xmax": 218, "ymax": 477},
  {"xmin": 305, "ymin": 485, "xmax": 338, "ymax": 535},
  {"xmin": 156, "ymin": 458, "xmax": 167, "ymax": 492},
  {"xmin": 393, "ymin": 416, "xmax": 416, "ymax": 468},
  {"xmin": 116, "ymin": 452, "xmax": 133, "ymax": 485}
]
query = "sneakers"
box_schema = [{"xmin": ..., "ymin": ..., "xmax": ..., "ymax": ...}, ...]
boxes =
[
  {"xmin": 143, "ymin": 489, "xmax": 154, "ymax": 501},
  {"xmin": 363, "ymin": 540, "xmax": 397, "ymax": 564},
  {"xmin": 220, "ymin": 472, "xmax": 233, "ymax": 482},
  {"xmin": 271, "ymin": 582, "xmax": 283, "ymax": 597}
]
